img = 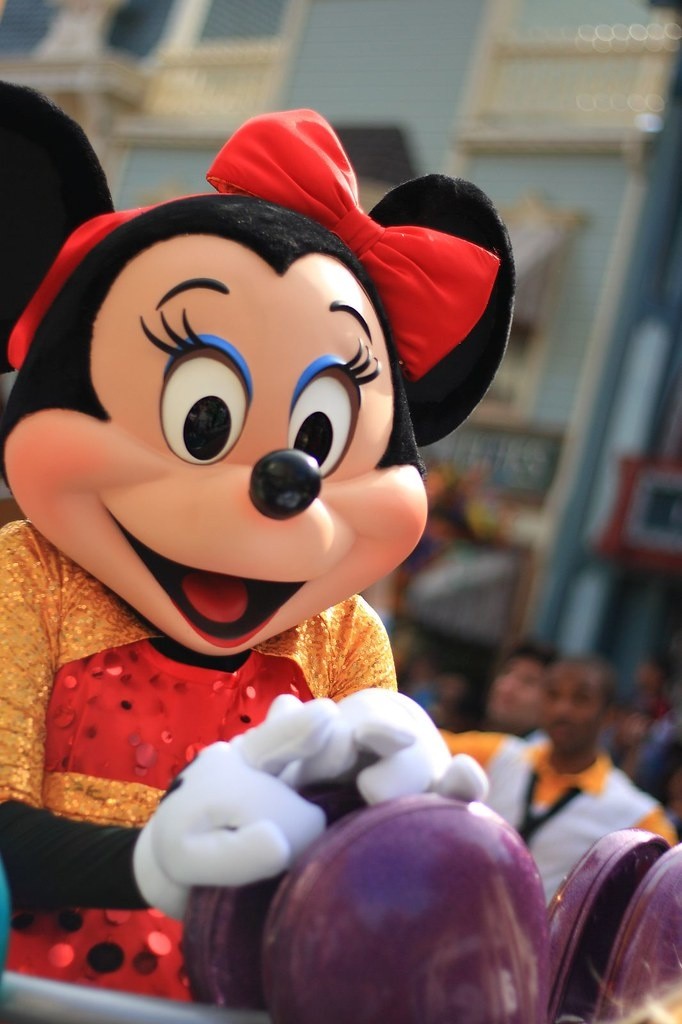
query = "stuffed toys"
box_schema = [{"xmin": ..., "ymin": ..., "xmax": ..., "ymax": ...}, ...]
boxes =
[{"xmin": 0, "ymin": 80, "xmax": 682, "ymax": 1024}]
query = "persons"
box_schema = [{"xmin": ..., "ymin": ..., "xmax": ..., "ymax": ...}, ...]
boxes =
[{"xmin": 383, "ymin": 613, "xmax": 681, "ymax": 903}]
